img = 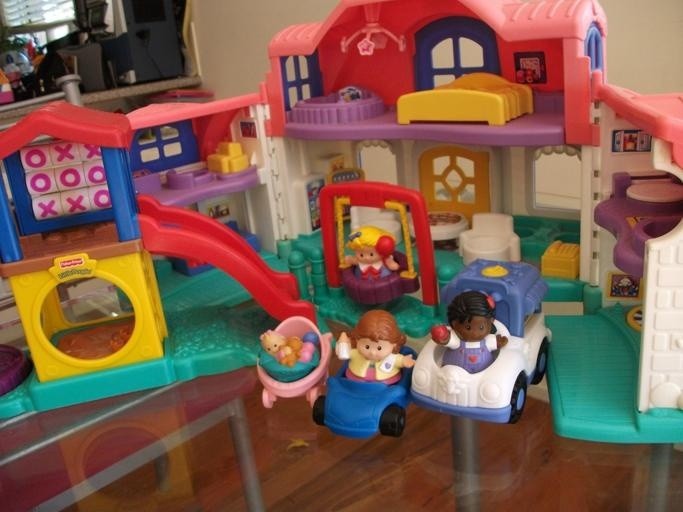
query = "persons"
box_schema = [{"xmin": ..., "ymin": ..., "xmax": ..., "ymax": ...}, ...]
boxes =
[
  {"xmin": 430, "ymin": 291, "xmax": 508, "ymax": 374},
  {"xmin": 341, "ymin": 226, "xmax": 400, "ymax": 282}
]
